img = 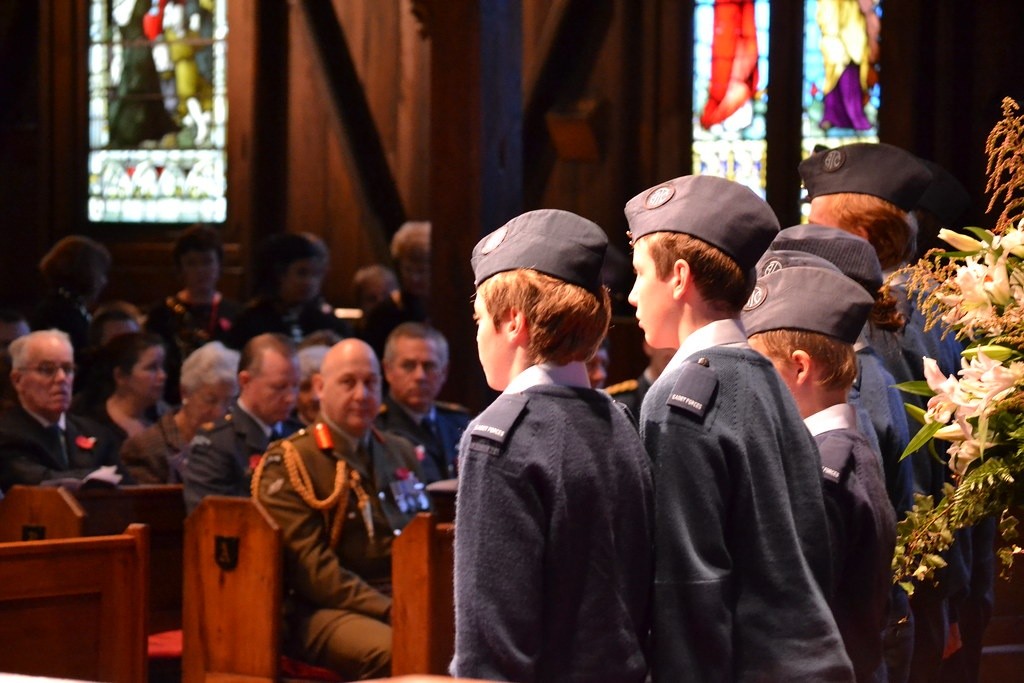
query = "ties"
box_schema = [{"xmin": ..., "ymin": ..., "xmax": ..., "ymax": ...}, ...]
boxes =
[
  {"xmin": 48, "ymin": 423, "xmax": 68, "ymax": 465},
  {"xmin": 356, "ymin": 441, "xmax": 372, "ymax": 469},
  {"xmin": 271, "ymin": 428, "xmax": 278, "ymax": 441},
  {"xmin": 421, "ymin": 417, "xmax": 440, "ymax": 445}
]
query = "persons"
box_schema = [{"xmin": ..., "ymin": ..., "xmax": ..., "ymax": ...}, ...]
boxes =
[
  {"xmin": 739, "ymin": 142, "xmax": 1000, "ymax": 683},
  {"xmin": 0, "ymin": 209, "xmax": 673, "ymax": 683},
  {"xmin": 623, "ymin": 175, "xmax": 858, "ymax": 683}
]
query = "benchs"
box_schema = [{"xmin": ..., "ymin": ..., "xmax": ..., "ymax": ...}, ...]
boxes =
[{"xmin": 0, "ymin": 483, "xmax": 461, "ymax": 683}]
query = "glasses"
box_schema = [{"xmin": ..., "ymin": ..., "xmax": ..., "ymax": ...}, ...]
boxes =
[{"xmin": 11, "ymin": 360, "xmax": 76, "ymax": 377}]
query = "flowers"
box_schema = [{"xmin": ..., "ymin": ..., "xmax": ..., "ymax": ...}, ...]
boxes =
[{"xmin": 878, "ymin": 94, "xmax": 1024, "ymax": 600}]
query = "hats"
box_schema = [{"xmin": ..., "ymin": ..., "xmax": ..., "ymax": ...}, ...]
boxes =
[
  {"xmin": 470, "ymin": 208, "xmax": 609, "ymax": 298},
  {"xmin": 623, "ymin": 174, "xmax": 781, "ymax": 280},
  {"xmin": 739, "ymin": 224, "xmax": 884, "ymax": 344},
  {"xmin": 797, "ymin": 143, "xmax": 934, "ymax": 214}
]
